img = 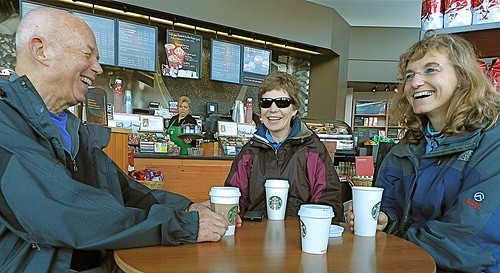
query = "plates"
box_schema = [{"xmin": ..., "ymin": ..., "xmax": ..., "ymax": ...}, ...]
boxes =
[{"xmin": 329, "ymin": 224, "xmax": 345, "ymax": 237}]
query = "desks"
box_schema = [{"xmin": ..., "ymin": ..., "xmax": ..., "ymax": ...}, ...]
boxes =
[{"xmin": 113, "ymin": 216, "xmax": 436, "ymax": 273}]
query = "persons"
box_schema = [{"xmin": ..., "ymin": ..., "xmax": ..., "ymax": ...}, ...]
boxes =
[
  {"xmin": 168, "ymin": 96, "xmax": 197, "ymax": 128},
  {"xmin": 0, "ymin": 7, "xmax": 242, "ymax": 273},
  {"xmin": 348, "ymin": 35, "xmax": 500, "ymax": 273},
  {"xmin": 224, "ymin": 71, "xmax": 344, "ymax": 221}
]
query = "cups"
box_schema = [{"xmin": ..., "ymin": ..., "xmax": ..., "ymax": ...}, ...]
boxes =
[
  {"xmin": 112, "ymin": 79, "xmax": 132, "ymax": 113},
  {"xmin": 231, "ymin": 97, "xmax": 252, "ymax": 123},
  {"xmin": 264, "ymin": 178, "xmax": 290, "ymax": 219},
  {"xmin": 164, "ymin": 42, "xmax": 185, "ymax": 71},
  {"xmin": 297, "ymin": 204, "xmax": 335, "ymax": 255},
  {"xmin": 208, "ymin": 186, "xmax": 242, "ymax": 236},
  {"xmin": 351, "ymin": 186, "xmax": 384, "ymax": 237}
]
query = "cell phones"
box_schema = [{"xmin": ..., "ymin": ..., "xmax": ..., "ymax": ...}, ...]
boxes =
[{"xmin": 243, "ymin": 211, "xmax": 264, "ymax": 221}]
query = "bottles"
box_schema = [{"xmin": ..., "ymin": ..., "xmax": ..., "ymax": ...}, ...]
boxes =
[{"xmin": 334, "ymin": 161, "xmax": 356, "ymax": 177}]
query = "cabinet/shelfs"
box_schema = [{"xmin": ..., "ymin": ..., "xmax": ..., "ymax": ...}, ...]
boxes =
[
  {"xmin": 350, "ymin": 98, "xmax": 407, "ymax": 143},
  {"xmin": 419, "ymin": 21, "xmax": 500, "ymax": 89}
]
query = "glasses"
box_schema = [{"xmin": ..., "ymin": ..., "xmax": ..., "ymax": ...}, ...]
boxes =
[
  {"xmin": 403, "ymin": 61, "xmax": 451, "ymax": 80},
  {"xmin": 258, "ymin": 97, "xmax": 294, "ymax": 108}
]
